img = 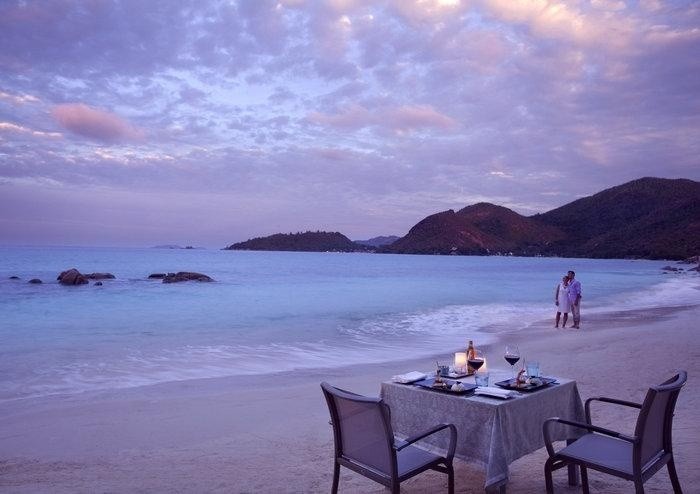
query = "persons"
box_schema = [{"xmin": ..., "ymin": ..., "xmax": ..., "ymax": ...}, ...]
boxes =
[
  {"xmin": 568, "ymin": 271, "xmax": 581, "ymax": 329},
  {"xmin": 554, "ymin": 276, "xmax": 583, "ymax": 330}
]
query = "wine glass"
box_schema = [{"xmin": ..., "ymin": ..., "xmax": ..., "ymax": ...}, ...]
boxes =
[{"xmin": 503, "ymin": 343, "xmax": 521, "ymax": 380}]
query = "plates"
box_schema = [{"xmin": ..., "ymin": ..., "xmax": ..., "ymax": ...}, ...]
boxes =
[
  {"xmin": 414, "ymin": 377, "xmax": 481, "ymax": 392},
  {"xmin": 494, "ymin": 375, "xmax": 557, "ymax": 390}
]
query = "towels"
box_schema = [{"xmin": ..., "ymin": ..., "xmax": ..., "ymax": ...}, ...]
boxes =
[
  {"xmin": 391, "ymin": 371, "xmax": 426, "ymax": 384},
  {"xmin": 474, "ymin": 386, "xmax": 511, "ymax": 399}
]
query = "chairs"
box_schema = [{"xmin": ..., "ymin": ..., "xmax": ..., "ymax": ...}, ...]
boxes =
[
  {"xmin": 320, "ymin": 382, "xmax": 457, "ymax": 494},
  {"xmin": 543, "ymin": 370, "xmax": 687, "ymax": 494}
]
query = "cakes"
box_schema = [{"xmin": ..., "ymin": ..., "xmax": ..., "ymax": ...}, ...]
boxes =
[{"xmin": 433, "ymin": 378, "xmax": 447, "ymax": 387}]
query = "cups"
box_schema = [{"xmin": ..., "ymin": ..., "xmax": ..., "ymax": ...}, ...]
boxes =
[
  {"xmin": 525, "ymin": 361, "xmax": 539, "ymax": 378},
  {"xmin": 467, "ymin": 350, "xmax": 490, "ymax": 387},
  {"xmin": 438, "ymin": 366, "xmax": 449, "ymax": 376}
]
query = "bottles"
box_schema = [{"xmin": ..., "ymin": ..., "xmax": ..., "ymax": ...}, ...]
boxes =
[{"xmin": 466, "ymin": 341, "xmax": 476, "ymax": 375}]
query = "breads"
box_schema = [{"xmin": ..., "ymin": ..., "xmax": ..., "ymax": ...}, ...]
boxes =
[
  {"xmin": 450, "ymin": 382, "xmax": 467, "ymax": 391},
  {"xmin": 525, "ymin": 376, "xmax": 543, "ymax": 388}
]
